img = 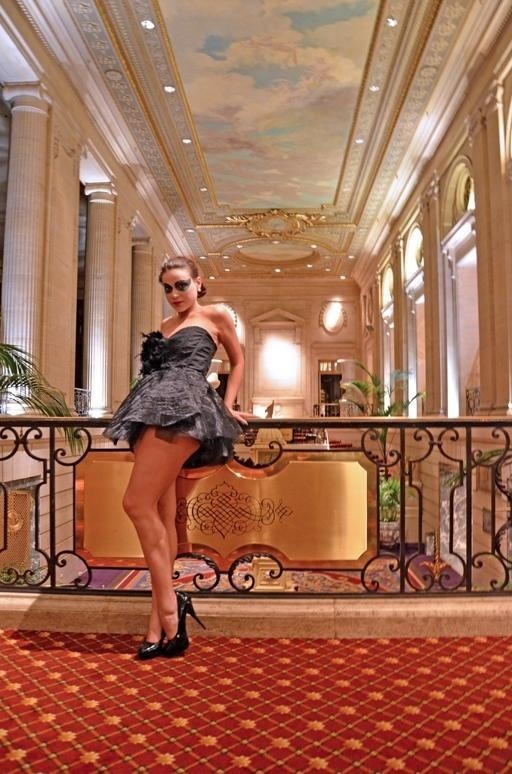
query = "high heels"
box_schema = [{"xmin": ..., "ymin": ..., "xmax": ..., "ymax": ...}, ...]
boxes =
[
  {"xmin": 139, "ymin": 624, "xmax": 166, "ymax": 660},
  {"xmin": 160, "ymin": 591, "xmax": 207, "ymax": 656}
]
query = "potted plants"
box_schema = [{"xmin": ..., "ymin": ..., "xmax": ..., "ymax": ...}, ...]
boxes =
[{"xmin": 338, "ymin": 361, "xmax": 428, "ymax": 546}]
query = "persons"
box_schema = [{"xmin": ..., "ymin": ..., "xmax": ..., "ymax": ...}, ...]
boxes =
[{"xmin": 100, "ymin": 254, "xmax": 260, "ymax": 661}]
